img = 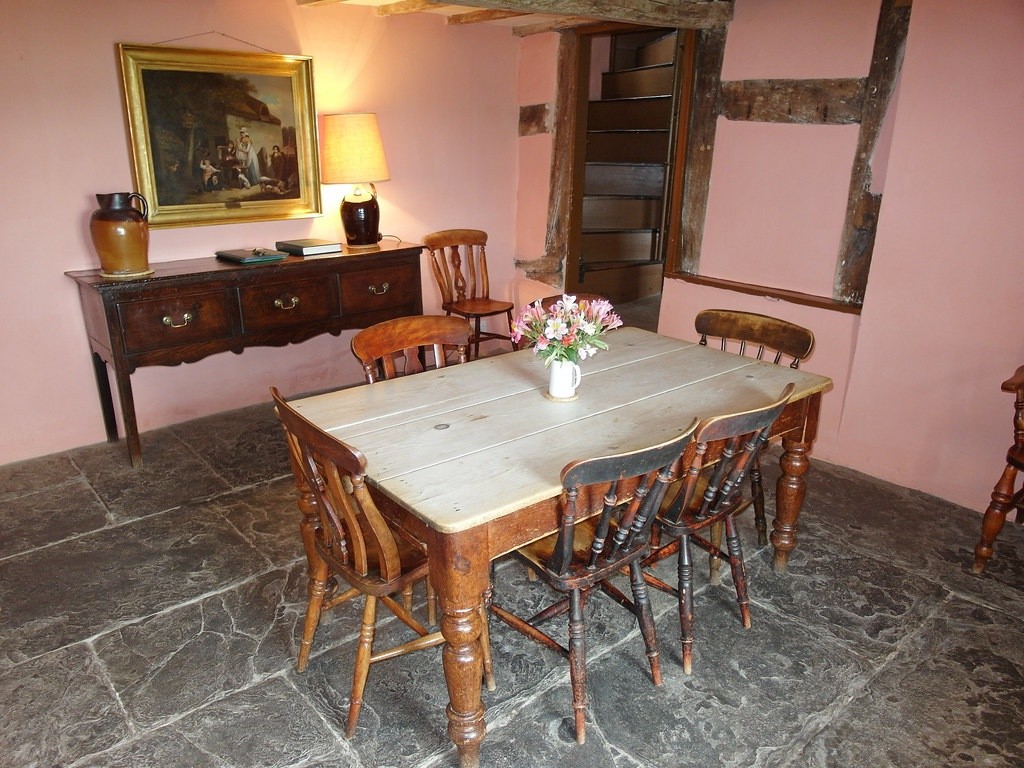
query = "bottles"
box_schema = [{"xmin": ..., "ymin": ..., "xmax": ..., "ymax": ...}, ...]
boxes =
[{"xmin": 89, "ymin": 192, "xmax": 149, "ymax": 275}]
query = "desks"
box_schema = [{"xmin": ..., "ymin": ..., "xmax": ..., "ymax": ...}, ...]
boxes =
[
  {"xmin": 65, "ymin": 234, "xmax": 428, "ymax": 467},
  {"xmin": 286, "ymin": 326, "xmax": 835, "ymax": 768}
]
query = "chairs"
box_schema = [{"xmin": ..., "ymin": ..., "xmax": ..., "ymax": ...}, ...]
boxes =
[
  {"xmin": 351, "ymin": 315, "xmax": 474, "ymax": 385},
  {"xmin": 694, "ymin": 309, "xmax": 815, "ymax": 369},
  {"xmin": 970, "ymin": 365, "xmax": 1023, "ymax": 574},
  {"xmin": 618, "ymin": 383, "xmax": 796, "ymax": 674},
  {"xmin": 491, "ymin": 415, "xmax": 701, "ymax": 745},
  {"xmin": 270, "ymin": 386, "xmax": 495, "ymax": 740},
  {"xmin": 424, "ymin": 229, "xmax": 518, "ymax": 358}
]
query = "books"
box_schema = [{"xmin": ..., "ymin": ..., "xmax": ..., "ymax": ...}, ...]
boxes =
[
  {"xmin": 214, "ymin": 247, "xmax": 289, "ymax": 265},
  {"xmin": 275, "ymin": 238, "xmax": 343, "ymax": 256}
]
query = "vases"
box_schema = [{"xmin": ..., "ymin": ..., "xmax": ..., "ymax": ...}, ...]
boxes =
[{"xmin": 549, "ymin": 360, "xmax": 582, "ymax": 398}]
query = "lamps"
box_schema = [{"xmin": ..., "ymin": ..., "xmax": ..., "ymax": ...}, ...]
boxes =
[{"xmin": 320, "ymin": 112, "xmax": 391, "ymax": 249}]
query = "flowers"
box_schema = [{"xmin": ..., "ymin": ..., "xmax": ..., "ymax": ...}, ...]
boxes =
[{"xmin": 508, "ymin": 293, "xmax": 623, "ymax": 368}]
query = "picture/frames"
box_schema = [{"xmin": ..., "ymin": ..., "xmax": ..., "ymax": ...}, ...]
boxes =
[{"xmin": 118, "ymin": 41, "xmax": 324, "ymax": 231}]
